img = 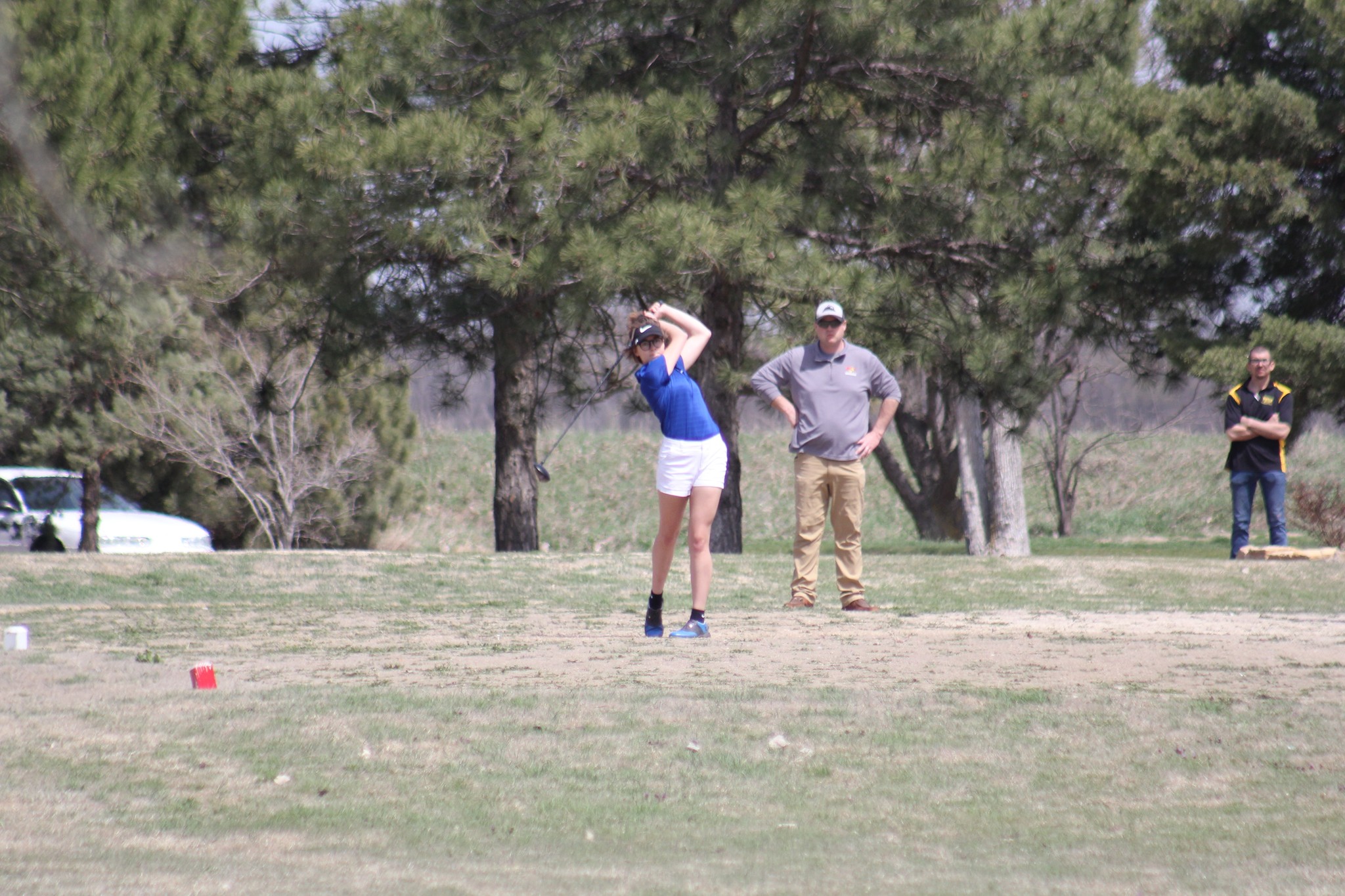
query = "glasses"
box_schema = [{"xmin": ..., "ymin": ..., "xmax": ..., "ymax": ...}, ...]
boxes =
[
  {"xmin": 638, "ymin": 337, "xmax": 663, "ymax": 350},
  {"xmin": 818, "ymin": 318, "xmax": 841, "ymax": 328},
  {"xmin": 1249, "ymin": 358, "xmax": 1270, "ymax": 366}
]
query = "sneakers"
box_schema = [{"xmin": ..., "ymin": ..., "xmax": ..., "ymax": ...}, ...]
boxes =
[
  {"xmin": 644, "ymin": 604, "xmax": 663, "ymax": 637},
  {"xmin": 669, "ymin": 620, "xmax": 711, "ymax": 638}
]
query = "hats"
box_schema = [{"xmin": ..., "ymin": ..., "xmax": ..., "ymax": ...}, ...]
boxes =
[
  {"xmin": 815, "ymin": 300, "xmax": 845, "ymax": 322},
  {"xmin": 632, "ymin": 325, "xmax": 664, "ymax": 346}
]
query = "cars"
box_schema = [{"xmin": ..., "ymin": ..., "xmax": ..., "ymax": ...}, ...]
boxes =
[{"xmin": 0, "ymin": 467, "xmax": 214, "ymax": 554}]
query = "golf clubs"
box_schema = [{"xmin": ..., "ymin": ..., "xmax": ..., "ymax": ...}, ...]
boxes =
[{"xmin": 531, "ymin": 298, "xmax": 664, "ymax": 482}]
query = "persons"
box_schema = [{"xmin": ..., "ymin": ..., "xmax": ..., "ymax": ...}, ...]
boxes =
[
  {"xmin": 629, "ymin": 302, "xmax": 729, "ymax": 637},
  {"xmin": 752, "ymin": 302, "xmax": 901, "ymax": 611},
  {"xmin": 1223, "ymin": 345, "xmax": 1295, "ymax": 559}
]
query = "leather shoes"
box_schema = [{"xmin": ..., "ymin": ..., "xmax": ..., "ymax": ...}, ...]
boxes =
[
  {"xmin": 842, "ymin": 599, "xmax": 879, "ymax": 611},
  {"xmin": 783, "ymin": 596, "xmax": 813, "ymax": 608}
]
